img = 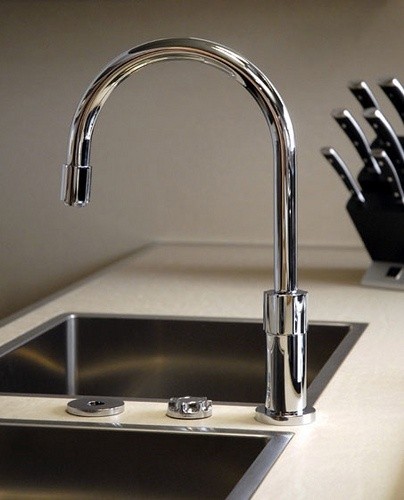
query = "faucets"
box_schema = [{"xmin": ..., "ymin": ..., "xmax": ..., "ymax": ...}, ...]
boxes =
[{"xmin": 60, "ymin": 34, "xmax": 298, "ymax": 293}]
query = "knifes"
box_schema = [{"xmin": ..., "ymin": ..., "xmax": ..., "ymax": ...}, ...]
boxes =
[{"xmin": 322, "ymin": 79, "xmax": 404, "ymax": 208}]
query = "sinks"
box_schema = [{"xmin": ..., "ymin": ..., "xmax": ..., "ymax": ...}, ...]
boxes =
[
  {"xmin": 0, "ymin": 315, "xmax": 349, "ymax": 406},
  {"xmin": 0, "ymin": 419, "xmax": 271, "ymax": 500}
]
